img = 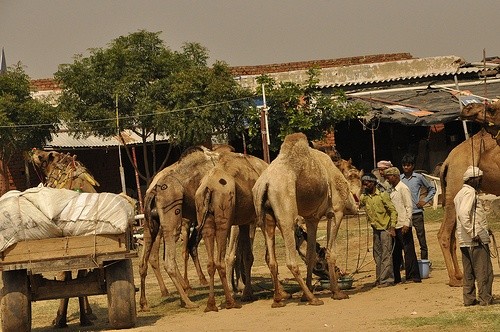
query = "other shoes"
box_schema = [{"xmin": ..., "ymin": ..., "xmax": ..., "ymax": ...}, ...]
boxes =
[
  {"xmin": 480, "ymin": 301, "xmax": 495, "ymax": 306},
  {"xmin": 463, "ymin": 298, "xmax": 477, "ymax": 306},
  {"xmin": 404, "ymin": 278, "xmax": 422, "ymax": 284},
  {"xmin": 372, "ymin": 279, "xmax": 395, "ymax": 288}
]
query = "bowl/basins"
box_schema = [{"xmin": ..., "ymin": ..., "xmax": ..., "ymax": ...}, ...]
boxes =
[{"xmin": 316, "ymin": 279, "xmax": 352, "ymax": 291}]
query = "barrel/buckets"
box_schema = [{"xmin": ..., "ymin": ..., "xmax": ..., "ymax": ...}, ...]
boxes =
[{"xmin": 417, "ymin": 260, "xmax": 432, "ymax": 279}]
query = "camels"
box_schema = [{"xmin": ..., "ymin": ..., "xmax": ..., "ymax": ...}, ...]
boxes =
[
  {"xmin": 252, "ymin": 133, "xmax": 364, "ymax": 309},
  {"xmin": 138, "ymin": 143, "xmax": 252, "ymax": 313},
  {"xmin": 439, "ymin": 98, "xmax": 500, "ymax": 287},
  {"xmin": 185, "ymin": 155, "xmax": 346, "ymax": 313}
]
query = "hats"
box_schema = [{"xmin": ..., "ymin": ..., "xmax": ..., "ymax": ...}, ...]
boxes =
[
  {"xmin": 376, "ymin": 160, "xmax": 393, "ymax": 168},
  {"xmin": 384, "ymin": 167, "xmax": 400, "ymax": 176},
  {"xmin": 463, "ymin": 165, "xmax": 483, "ymax": 181}
]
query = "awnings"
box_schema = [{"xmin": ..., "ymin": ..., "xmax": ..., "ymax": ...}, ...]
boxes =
[{"xmin": 352, "ymin": 82, "xmax": 500, "ymax": 128}]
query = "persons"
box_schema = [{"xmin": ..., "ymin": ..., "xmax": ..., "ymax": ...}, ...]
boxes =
[
  {"xmin": 351, "ymin": 154, "xmax": 436, "ymax": 288},
  {"xmin": 453, "ymin": 166, "xmax": 500, "ymax": 306}
]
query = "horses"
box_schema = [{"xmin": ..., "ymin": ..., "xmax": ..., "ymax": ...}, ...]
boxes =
[{"xmin": 31, "ymin": 148, "xmax": 98, "ymax": 326}]
